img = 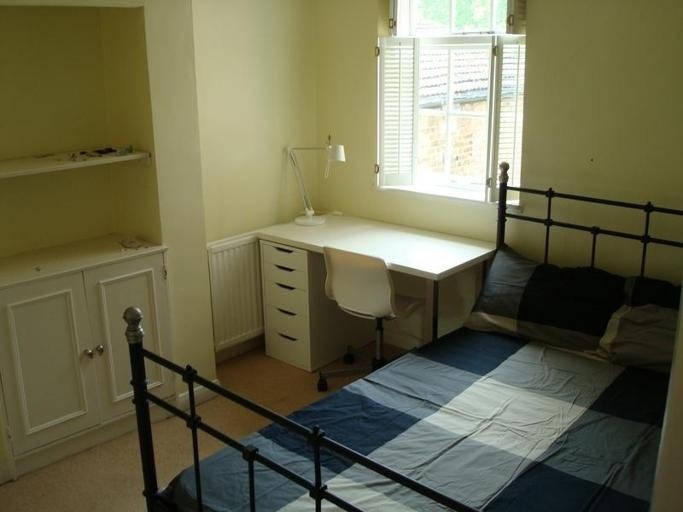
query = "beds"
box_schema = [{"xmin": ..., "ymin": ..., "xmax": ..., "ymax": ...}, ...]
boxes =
[{"xmin": 121, "ymin": 162, "xmax": 682, "ymax": 512}]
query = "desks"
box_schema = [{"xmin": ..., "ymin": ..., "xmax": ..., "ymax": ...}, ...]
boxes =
[{"xmin": 260, "ymin": 212, "xmax": 498, "ymax": 373}]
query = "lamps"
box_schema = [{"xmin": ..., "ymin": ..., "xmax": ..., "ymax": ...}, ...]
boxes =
[{"xmin": 282, "ymin": 137, "xmax": 347, "ymax": 226}]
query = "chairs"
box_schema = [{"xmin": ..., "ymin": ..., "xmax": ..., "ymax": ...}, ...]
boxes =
[{"xmin": 317, "ymin": 246, "xmax": 429, "ymax": 394}]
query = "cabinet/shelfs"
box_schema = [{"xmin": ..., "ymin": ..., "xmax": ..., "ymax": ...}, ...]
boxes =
[{"xmin": 257, "ymin": 239, "xmax": 377, "ymax": 374}]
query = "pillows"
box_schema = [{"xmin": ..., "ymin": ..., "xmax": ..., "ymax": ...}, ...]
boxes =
[{"xmin": 462, "ymin": 241, "xmax": 681, "ymax": 374}]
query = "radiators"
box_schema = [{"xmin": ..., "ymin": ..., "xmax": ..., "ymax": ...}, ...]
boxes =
[{"xmin": 204, "ymin": 230, "xmax": 273, "ymax": 353}]
